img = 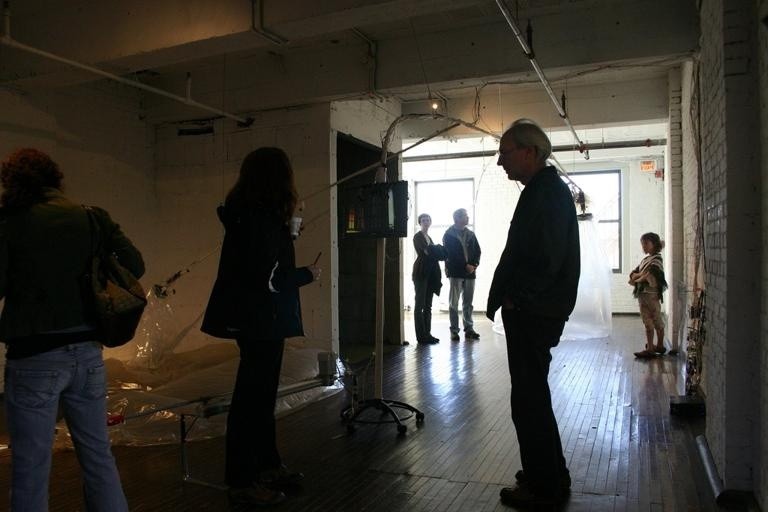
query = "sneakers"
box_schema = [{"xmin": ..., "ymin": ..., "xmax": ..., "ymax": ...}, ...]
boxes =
[
  {"xmin": 451, "ymin": 332, "xmax": 460, "ymax": 340},
  {"xmin": 418, "ymin": 335, "xmax": 439, "ymax": 343},
  {"xmin": 500, "ymin": 467, "xmax": 570, "ymax": 508},
  {"xmin": 465, "ymin": 328, "xmax": 480, "ymax": 338},
  {"xmin": 229, "ymin": 461, "xmax": 306, "ymax": 506},
  {"xmin": 633, "ymin": 342, "xmax": 666, "ymax": 358}
]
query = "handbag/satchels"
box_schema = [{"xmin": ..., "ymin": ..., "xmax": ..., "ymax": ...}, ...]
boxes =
[{"xmin": 90, "ymin": 250, "xmax": 148, "ymax": 347}]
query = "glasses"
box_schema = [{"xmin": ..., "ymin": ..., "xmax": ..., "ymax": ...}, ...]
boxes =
[{"xmin": 499, "ymin": 143, "xmax": 528, "ymax": 159}]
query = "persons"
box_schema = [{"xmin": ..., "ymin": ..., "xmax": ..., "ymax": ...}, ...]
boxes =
[
  {"xmin": 444, "ymin": 209, "xmax": 481, "ymax": 341},
  {"xmin": 412, "ymin": 214, "xmax": 440, "ymax": 347},
  {"xmin": 0, "ymin": 145, "xmax": 146, "ymax": 510},
  {"xmin": 482, "ymin": 116, "xmax": 579, "ymax": 509},
  {"xmin": 627, "ymin": 233, "xmax": 666, "ymax": 359},
  {"xmin": 200, "ymin": 146, "xmax": 323, "ymax": 488}
]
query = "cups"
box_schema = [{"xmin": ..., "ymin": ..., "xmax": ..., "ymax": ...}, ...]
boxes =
[{"xmin": 289, "ymin": 217, "xmax": 303, "ymax": 236}]
